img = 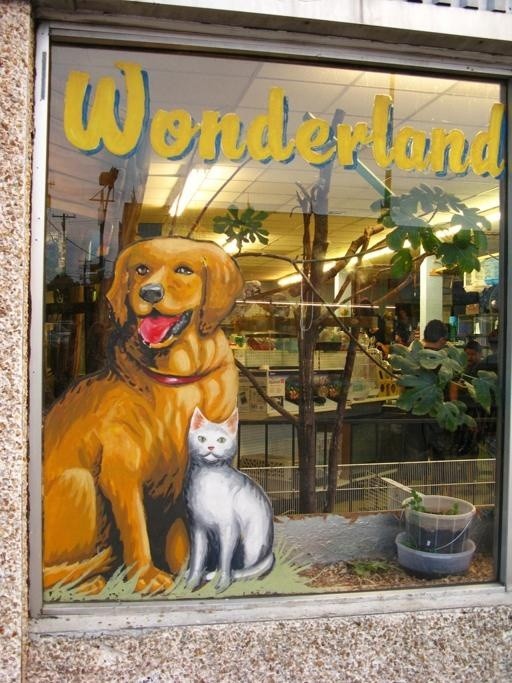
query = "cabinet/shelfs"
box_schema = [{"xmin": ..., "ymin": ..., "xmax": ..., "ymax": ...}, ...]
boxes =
[{"xmin": 231, "ymin": 294, "xmax": 498, "ymax": 476}]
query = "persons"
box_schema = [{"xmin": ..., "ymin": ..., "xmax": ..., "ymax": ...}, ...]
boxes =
[{"xmin": 362, "ymin": 301, "xmax": 498, "ymax": 458}]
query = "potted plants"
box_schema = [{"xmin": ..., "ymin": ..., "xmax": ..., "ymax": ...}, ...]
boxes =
[{"xmin": 394, "ymin": 488, "xmax": 479, "ymax": 580}]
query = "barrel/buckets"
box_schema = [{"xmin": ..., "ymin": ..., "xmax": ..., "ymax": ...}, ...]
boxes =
[{"xmin": 398, "ymin": 494, "xmax": 476, "ymax": 554}]
